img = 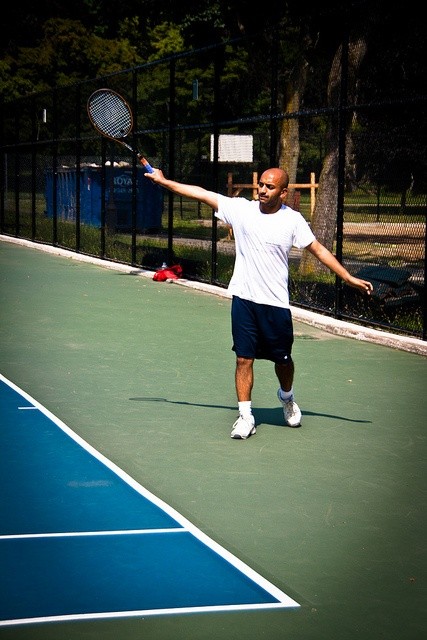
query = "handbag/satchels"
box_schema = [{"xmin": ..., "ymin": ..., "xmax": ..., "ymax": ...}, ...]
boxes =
[{"xmin": 154, "ymin": 266, "xmax": 182, "ymax": 282}]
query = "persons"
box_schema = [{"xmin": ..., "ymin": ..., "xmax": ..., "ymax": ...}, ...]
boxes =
[{"xmin": 143, "ymin": 168, "xmax": 373, "ymax": 440}]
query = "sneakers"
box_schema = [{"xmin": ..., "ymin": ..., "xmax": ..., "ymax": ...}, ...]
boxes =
[
  {"xmin": 230, "ymin": 416, "xmax": 256, "ymax": 439},
  {"xmin": 277, "ymin": 389, "xmax": 302, "ymax": 427}
]
